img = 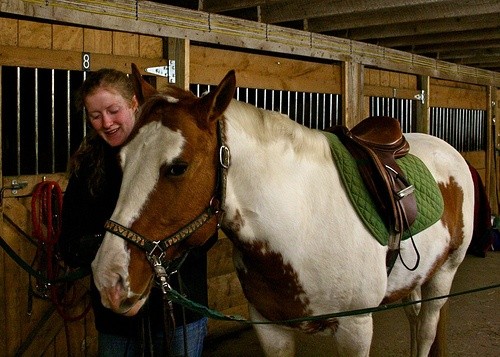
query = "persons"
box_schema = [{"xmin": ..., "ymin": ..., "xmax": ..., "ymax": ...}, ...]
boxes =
[{"xmin": 60, "ymin": 68, "xmax": 220, "ymax": 357}]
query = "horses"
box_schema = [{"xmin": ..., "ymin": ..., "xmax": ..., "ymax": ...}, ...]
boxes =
[{"xmin": 90, "ymin": 63, "xmax": 475, "ymax": 357}]
